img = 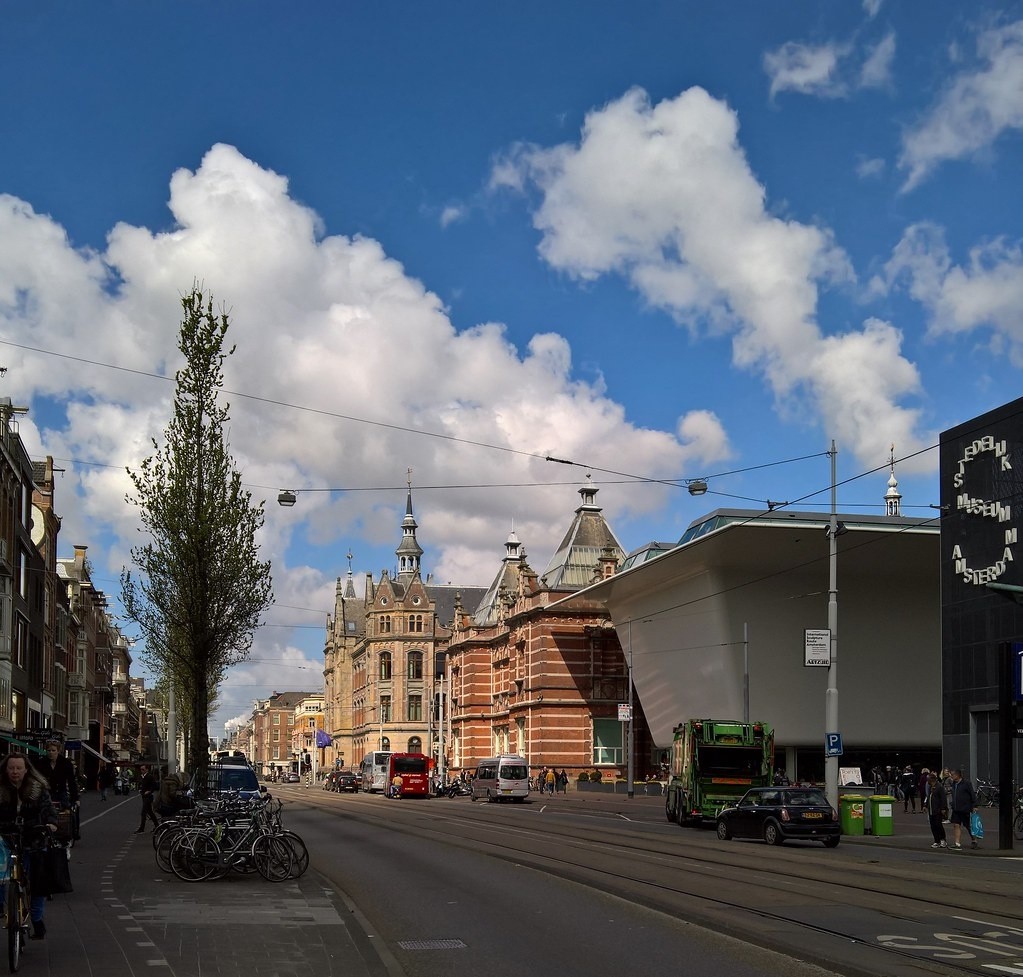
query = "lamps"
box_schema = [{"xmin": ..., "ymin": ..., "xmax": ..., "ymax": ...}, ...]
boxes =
[
  {"xmin": 688, "ymin": 480, "xmax": 708, "ymax": 496},
  {"xmin": 277, "ymin": 490, "xmax": 296, "ymax": 506}
]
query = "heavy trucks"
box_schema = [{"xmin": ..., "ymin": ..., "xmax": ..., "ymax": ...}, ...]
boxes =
[{"xmin": 666, "ymin": 718, "xmax": 785, "ymax": 828}]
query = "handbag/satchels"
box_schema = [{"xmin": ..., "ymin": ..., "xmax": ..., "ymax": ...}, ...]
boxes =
[
  {"xmin": 22, "ymin": 825, "xmax": 76, "ymax": 898},
  {"xmin": 970, "ymin": 811, "xmax": 984, "ymax": 840}
]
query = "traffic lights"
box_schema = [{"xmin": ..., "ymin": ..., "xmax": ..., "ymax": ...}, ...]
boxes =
[{"xmin": 306, "ymin": 754, "xmax": 310, "ymax": 764}]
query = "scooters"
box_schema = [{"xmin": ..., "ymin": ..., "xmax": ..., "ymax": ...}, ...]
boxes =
[
  {"xmin": 116, "ymin": 776, "xmax": 131, "ymax": 796},
  {"xmin": 448, "ymin": 782, "xmax": 473, "ymax": 799}
]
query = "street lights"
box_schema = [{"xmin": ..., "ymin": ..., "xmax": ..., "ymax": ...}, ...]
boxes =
[{"xmin": 825, "ymin": 520, "xmax": 850, "ymax": 816}]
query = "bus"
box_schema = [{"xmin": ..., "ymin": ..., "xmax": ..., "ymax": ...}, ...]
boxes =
[
  {"xmin": 359, "ymin": 751, "xmax": 398, "ymax": 794},
  {"xmin": 381, "ymin": 753, "xmax": 430, "ymax": 799}
]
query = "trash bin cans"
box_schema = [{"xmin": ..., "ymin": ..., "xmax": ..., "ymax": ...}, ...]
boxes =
[
  {"xmin": 870, "ymin": 795, "xmax": 896, "ymax": 836},
  {"xmin": 840, "ymin": 793, "xmax": 866, "ymax": 836}
]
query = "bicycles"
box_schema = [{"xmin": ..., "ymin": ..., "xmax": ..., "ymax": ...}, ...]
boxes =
[
  {"xmin": 973, "ymin": 777, "xmax": 1023, "ymax": 841},
  {"xmin": 152, "ymin": 786, "xmax": 310, "ymax": 883},
  {"xmin": 0, "ymin": 816, "xmax": 56, "ymax": 974}
]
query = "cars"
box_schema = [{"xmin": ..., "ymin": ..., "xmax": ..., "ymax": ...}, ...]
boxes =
[
  {"xmin": 281, "ymin": 773, "xmax": 300, "ymax": 783},
  {"xmin": 321, "ymin": 771, "xmax": 362, "ymax": 793},
  {"xmin": 714, "ymin": 786, "xmax": 842, "ymax": 848}
]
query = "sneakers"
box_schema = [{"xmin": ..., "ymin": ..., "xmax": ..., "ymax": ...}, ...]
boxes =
[
  {"xmin": 134, "ymin": 830, "xmax": 145, "ymax": 834},
  {"xmin": 939, "ymin": 840, "xmax": 947, "ymax": 848},
  {"xmin": 970, "ymin": 841, "xmax": 977, "ymax": 849},
  {"xmin": 947, "ymin": 844, "xmax": 963, "ymax": 851},
  {"xmin": 930, "ymin": 843, "xmax": 939, "ymax": 848}
]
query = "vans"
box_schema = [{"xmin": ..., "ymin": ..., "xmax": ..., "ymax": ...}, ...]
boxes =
[{"xmin": 471, "ymin": 755, "xmax": 529, "ymax": 803}]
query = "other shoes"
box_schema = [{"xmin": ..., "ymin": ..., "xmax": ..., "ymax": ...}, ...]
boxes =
[
  {"xmin": 32, "ymin": 919, "xmax": 46, "ymax": 941},
  {"xmin": 941, "ymin": 820, "xmax": 951, "ymax": 824}
]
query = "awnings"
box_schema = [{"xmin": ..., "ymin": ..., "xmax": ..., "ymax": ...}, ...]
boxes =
[
  {"xmin": 0, "ymin": 736, "xmax": 48, "ymax": 754},
  {"xmin": 106, "ymin": 744, "xmax": 119, "ymax": 757},
  {"xmin": 79, "ymin": 742, "xmax": 111, "ymax": 763}
]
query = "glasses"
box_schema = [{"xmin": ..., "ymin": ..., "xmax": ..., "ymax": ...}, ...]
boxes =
[{"xmin": 45, "ymin": 740, "xmax": 59, "ymax": 744}]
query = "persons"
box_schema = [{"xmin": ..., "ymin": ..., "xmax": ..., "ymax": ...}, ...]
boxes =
[
  {"xmin": 303, "ymin": 770, "xmax": 324, "ymax": 781},
  {"xmin": 34, "ymin": 738, "xmax": 81, "ymax": 860},
  {"xmin": 773, "ymin": 766, "xmax": 791, "ymax": 786},
  {"xmin": 111, "ymin": 766, "xmax": 135, "ymax": 791},
  {"xmin": 460, "ymin": 768, "xmax": 471, "ymax": 784},
  {"xmin": 949, "ymin": 768, "xmax": 977, "ymax": 850},
  {"xmin": 923, "ymin": 774, "xmax": 948, "ymax": 848},
  {"xmin": 870, "ymin": 764, "xmax": 953, "ymax": 824},
  {"xmin": 134, "ymin": 763, "xmax": 160, "ymax": 834},
  {"xmin": 97, "ymin": 766, "xmax": 110, "ymax": 801},
  {"xmin": 391, "ymin": 773, "xmax": 403, "ymax": 797},
  {"xmin": 538, "ymin": 766, "xmax": 569, "ymax": 797},
  {"xmin": 271, "ymin": 769, "xmax": 277, "ymax": 784},
  {"xmin": 0, "ymin": 751, "xmax": 57, "ymax": 940}
]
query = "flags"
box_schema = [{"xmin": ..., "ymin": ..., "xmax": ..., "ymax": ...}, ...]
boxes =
[{"xmin": 317, "ymin": 728, "xmax": 331, "ymax": 747}]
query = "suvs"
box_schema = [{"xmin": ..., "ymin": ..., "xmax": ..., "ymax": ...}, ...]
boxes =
[{"xmin": 185, "ymin": 764, "xmax": 268, "ymax": 798}]
